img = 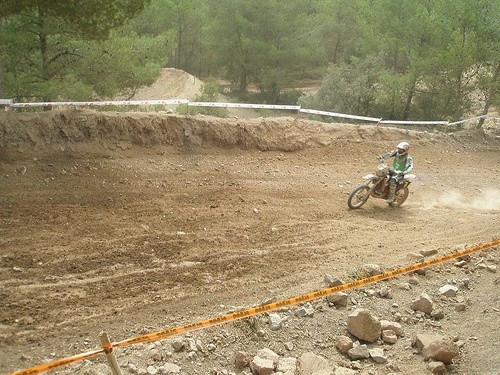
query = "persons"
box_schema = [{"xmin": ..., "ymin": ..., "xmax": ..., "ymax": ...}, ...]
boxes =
[{"xmin": 377, "ymin": 141, "xmax": 413, "ymax": 203}]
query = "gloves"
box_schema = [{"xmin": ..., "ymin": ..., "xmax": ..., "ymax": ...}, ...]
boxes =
[{"xmin": 378, "ymin": 155, "xmax": 384, "ymax": 160}]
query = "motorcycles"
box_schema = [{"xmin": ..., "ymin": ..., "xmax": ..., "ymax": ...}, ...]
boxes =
[{"xmin": 348, "ymin": 155, "xmax": 416, "ymax": 210}]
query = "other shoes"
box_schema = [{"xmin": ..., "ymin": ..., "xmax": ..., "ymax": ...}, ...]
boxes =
[{"xmin": 386, "ymin": 196, "xmax": 395, "ymax": 203}]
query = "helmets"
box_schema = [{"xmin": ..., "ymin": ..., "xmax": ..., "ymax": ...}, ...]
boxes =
[{"xmin": 396, "ymin": 142, "xmax": 410, "ymax": 157}]
